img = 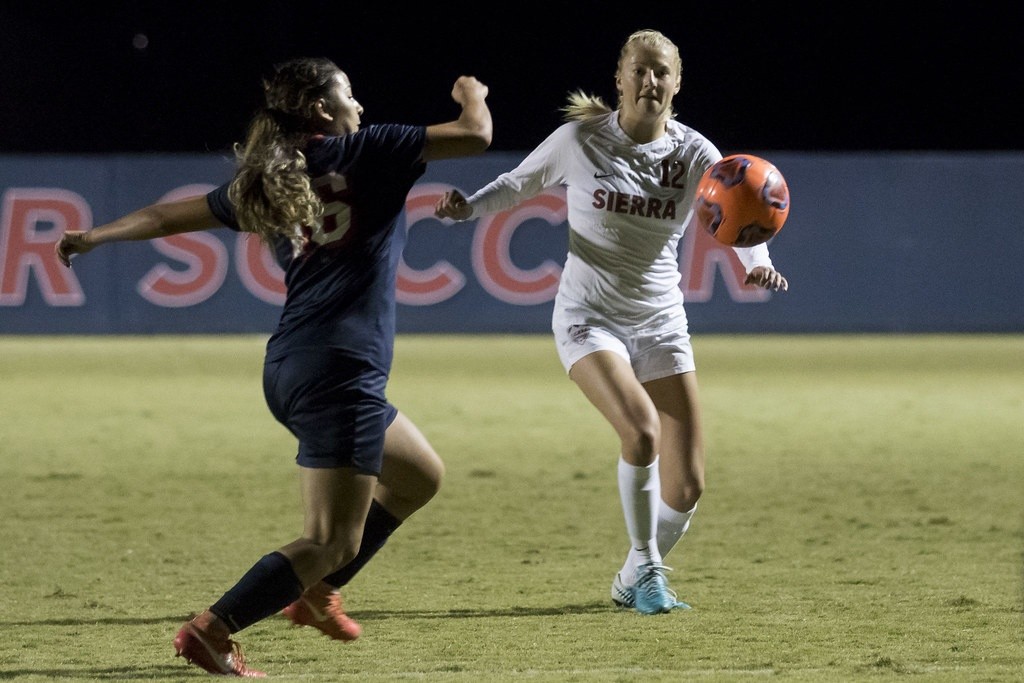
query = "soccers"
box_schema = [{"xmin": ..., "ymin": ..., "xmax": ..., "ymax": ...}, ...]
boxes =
[{"xmin": 693, "ymin": 154, "xmax": 792, "ymax": 249}]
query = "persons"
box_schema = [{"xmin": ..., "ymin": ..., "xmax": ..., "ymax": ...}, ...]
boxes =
[
  {"xmin": 436, "ymin": 31, "xmax": 788, "ymax": 614},
  {"xmin": 55, "ymin": 59, "xmax": 493, "ymax": 678}
]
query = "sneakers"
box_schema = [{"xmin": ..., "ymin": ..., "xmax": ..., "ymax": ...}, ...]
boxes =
[
  {"xmin": 171, "ymin": 613, "xmax": 269, "ymax": 678},
  {"xmin": 610, "ymin": 559, "xmax": 691, "ymax": 614},
  {"xmin": 282, "ymin": 586, "xmax": 362, "ymax": 645}
]
